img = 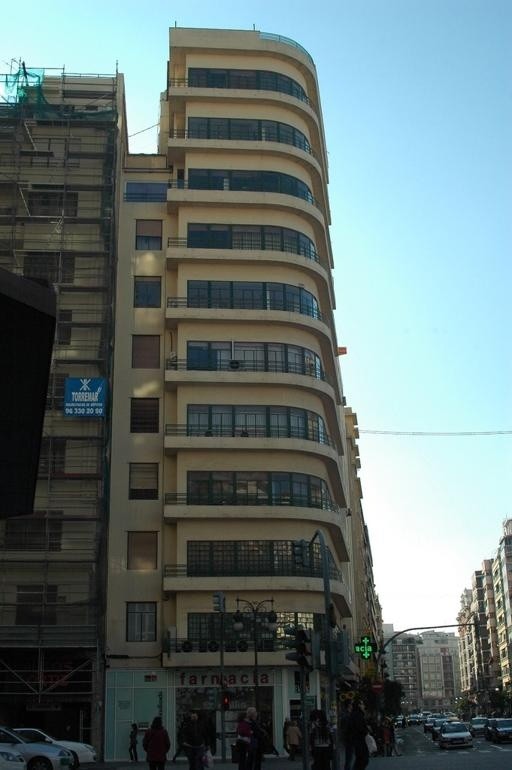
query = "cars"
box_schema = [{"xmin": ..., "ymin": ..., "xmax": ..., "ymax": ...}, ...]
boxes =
[
  {"xmin": 0, "ymin": 726, "xmax": 100, "ymax": 770},
  {"xmin": 393, "ymin": 709, "xmax": 511, "ymax": 749}
]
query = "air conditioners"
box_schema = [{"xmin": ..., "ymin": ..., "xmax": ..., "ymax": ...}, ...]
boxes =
[
  {"xmin": 54, "ymin": 593, "xmax": 71, "ymax": 606},
  {"xmin": 226, "ymin": 358, "xmax": 246, "ymax": 371},
  {"xmin": 199, "ymin": 427, "xmax": 217, "ymax": 437},
  {"xmin": 234, "ymin": 427, "xmax": 251, "ymax": 437}
]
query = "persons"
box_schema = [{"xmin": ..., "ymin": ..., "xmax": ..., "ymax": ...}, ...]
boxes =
[
  {"xmin": 171, "ymin": 708, "xmax": 212, "ymax": 770},
  {"xmin": 128, "ymin": 723, "xmax": 139, "ymax": 764},
  {"xmin": 457, "ymin": 708, "xmax": 508, "ymax": 722},
  {"xmin": 231, "ymin": 707, "xmax": 273, "ymax": 769},
  {"xmin": 281, "ymin": 701, "xmax": 409, "ymax": 770},
  {"xmin": 142, "ymin": 716, "xmax": 171, "ymax": 770}
]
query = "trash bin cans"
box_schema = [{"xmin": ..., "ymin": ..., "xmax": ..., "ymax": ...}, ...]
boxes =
[{"xmin": 230, "ymin": 744, "xmax": 246, "ymax": 763}]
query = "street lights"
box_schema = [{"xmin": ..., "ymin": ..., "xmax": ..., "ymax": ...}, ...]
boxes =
[{"xmin": 233, "ymin": 597, "xmax": 278, "ymax": 707}]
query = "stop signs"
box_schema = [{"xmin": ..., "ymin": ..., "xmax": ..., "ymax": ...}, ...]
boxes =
[{"xmin": 372, "ymin": 681, "xmax": 383, "ymax": 693}]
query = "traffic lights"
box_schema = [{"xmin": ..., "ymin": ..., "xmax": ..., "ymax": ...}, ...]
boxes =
[
  {"xmin": 223, "ymin": 697, "xmax": 230, "ymax": 710},
  {"xmin": 282, "ymin": 626, "xmax": 298, "ymax": 662},
  {"xmin": 475, "ymin": 621, "xmax": 480, "ymax": 632},
  {"xmin": 299, "ymin": 628, "xmax": 313, "ymax": 669},
  {"xmin": 212, "ymin": 591, "xmax": 226, "ymax": 615}
]
